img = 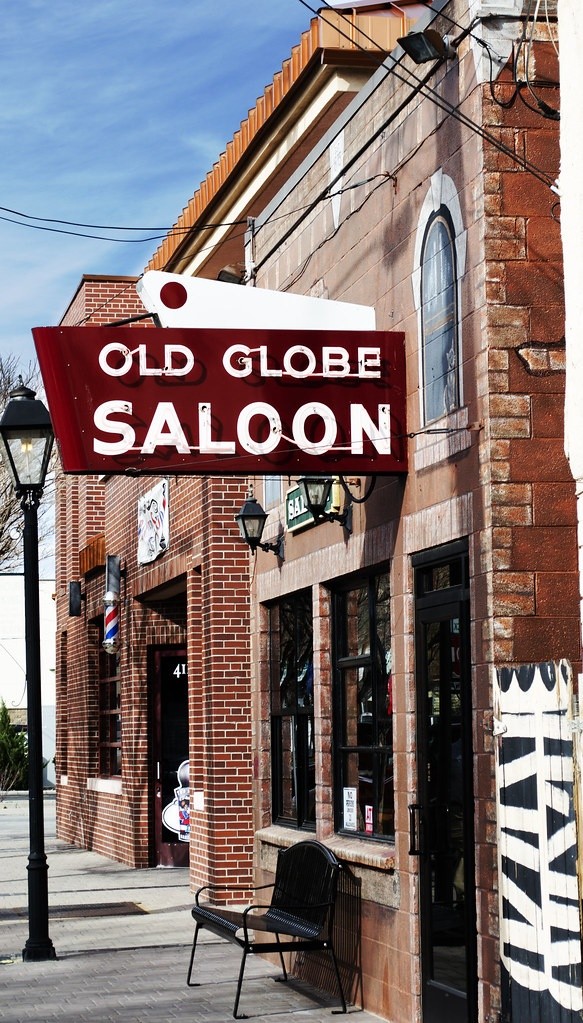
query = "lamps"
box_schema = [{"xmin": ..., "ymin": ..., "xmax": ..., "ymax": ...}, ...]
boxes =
[
  {"xmin": 106, "ymin": 555, "xmax": 126, "ymax": 594},
  {"xmin": 234, "ymin": 490, "xmax": 285, "ymax": 561},
  {"xmin": 396, "ymin": 28, "xmax": 456, "ymax": 65},
  {"xmin": 69, "ymin": 581, "xmax": 86, "ymax": 617},
  {"xmin": 296, "ymin": 476, "xmax": 353, "ymax": 534}
]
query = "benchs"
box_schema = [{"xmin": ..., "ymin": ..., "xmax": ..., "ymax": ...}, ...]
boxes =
[{"xmin": 187, "ymin": 840, "xmax": 347, "ymax": 1020}]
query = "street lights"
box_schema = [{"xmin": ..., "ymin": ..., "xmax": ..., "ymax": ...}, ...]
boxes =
[{"xmin": 1, "ymin": 374, "xmax": 69, "ymax": 965}]
